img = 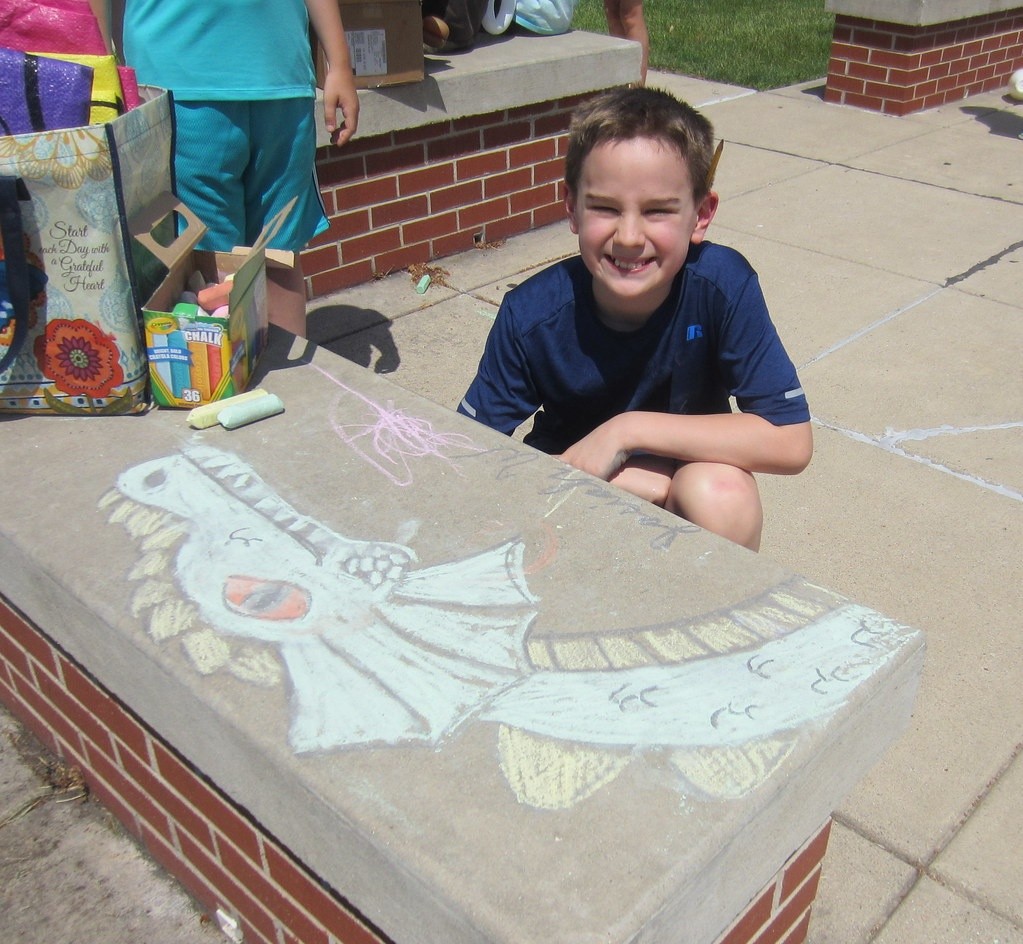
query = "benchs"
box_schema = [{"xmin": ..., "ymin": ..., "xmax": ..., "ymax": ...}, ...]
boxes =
[
  {"xmin": 264, "ymin": 23, "xmax": 643, "ymax": 344},
  {"xmin": 822, "ymin": 0, "xmax": 1023, "ymax": 117},
  {"xmin": 0, "ymin": 333, "xmax": 930, "ymax": 944}
]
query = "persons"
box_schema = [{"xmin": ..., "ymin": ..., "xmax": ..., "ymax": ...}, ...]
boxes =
[
  {"xmin": 90, "ymin": 0, "xmax": 360, "ymax": 341},
  {"xmin": 604, "ymin": 0, "xmax": 649, "ymax": 86},
  {"xmin": 455, "ymin": 86, "xmax": 813, "ymax": 553}
]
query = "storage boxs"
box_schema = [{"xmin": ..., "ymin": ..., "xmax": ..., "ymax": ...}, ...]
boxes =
[
  {"xmin": 309, "ymin": 0, "xmax": 424, "ymax": 91},
  {"xmin": 118, "ymin": 195, "xmax": 303, "ymax": 404}
]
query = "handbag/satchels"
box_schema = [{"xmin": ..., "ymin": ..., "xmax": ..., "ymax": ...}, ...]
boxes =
[{"xmin": 0, "ymin": 82, "xmax": 173, "ymax": 416}]
